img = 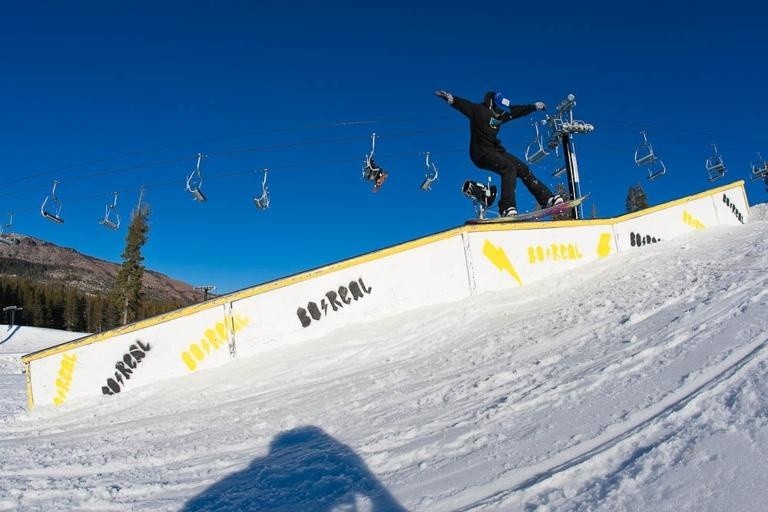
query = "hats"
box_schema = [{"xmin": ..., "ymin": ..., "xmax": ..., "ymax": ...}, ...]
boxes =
[{"xmin": 494, "ymin": 92, "xmax": 511, "ymax": 115}]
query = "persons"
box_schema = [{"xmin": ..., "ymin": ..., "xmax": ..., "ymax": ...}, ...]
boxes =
[{"xmin": 435, "ymin": 91, "xmax": 564, "ymax": 218}]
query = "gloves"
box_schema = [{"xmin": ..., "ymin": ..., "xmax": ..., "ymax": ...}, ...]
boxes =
[
  {"xmin": 435, "ymin": 89, "xmax": 454, "ymax": 105},
  {"xmin": 535, "ymin": 102, "xmax": 546, "ymax": 112}
]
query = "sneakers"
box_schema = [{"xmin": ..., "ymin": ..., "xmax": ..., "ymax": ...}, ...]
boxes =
[
  {"xmin": 501, "ymin": 207, "xmax": 518, "ymax": 217},
  {"xmin": 540, "ymin": 195, "xmax": 564, "ymax": 210}
]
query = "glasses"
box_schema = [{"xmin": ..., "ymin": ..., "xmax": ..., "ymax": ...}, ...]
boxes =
[{"xmin": 492, "ymin": 102, "xmax": 504, "ymax": 114}]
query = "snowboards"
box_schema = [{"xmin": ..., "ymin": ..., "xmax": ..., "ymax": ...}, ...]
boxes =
[{"xmin": 465, "ymin": 197, "xmax": 586, "ymax": 224}]
